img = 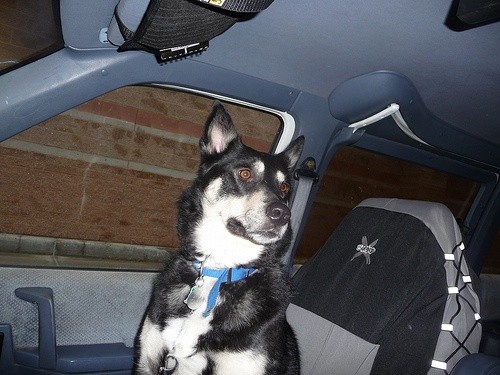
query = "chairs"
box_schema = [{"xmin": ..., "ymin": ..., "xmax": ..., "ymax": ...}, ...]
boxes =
[{"xmin": 286, "ymin": 198, "xmax": 483, "ymax": 375}]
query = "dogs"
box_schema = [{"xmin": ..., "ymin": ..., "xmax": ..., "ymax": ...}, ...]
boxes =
[{"xmin": 131, "ymin": 102, "xmax": 304, "ymax": 375}]
